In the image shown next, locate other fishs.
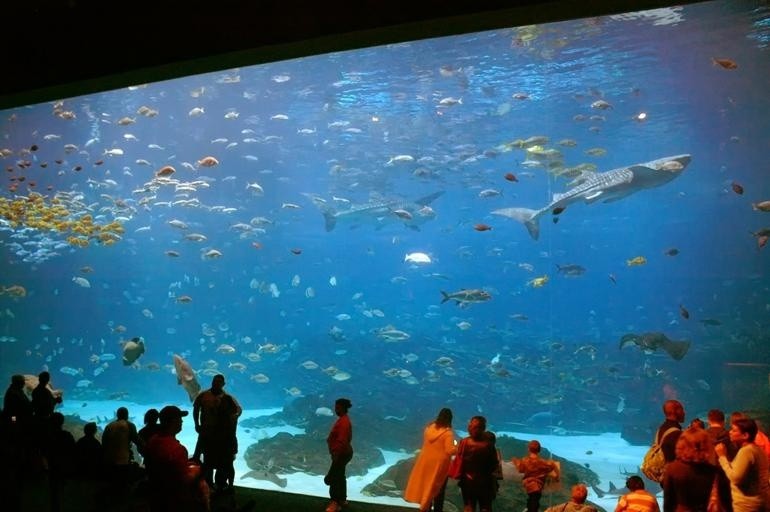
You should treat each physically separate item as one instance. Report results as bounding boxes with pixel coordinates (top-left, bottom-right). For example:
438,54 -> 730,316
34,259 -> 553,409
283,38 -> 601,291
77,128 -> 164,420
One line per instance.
0,0 -> 770,512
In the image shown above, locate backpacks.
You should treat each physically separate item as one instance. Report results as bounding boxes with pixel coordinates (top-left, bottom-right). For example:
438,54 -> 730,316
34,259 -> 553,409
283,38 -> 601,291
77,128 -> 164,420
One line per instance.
641,426 -> 681,482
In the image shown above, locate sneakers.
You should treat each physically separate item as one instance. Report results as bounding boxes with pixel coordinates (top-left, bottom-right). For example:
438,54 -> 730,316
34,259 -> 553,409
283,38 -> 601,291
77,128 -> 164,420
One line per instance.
326,500 -> 342,512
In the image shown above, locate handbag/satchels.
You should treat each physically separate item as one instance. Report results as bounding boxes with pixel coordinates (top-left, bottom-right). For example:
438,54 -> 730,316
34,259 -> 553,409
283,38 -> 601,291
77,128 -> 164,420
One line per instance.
446,457 -> 463,480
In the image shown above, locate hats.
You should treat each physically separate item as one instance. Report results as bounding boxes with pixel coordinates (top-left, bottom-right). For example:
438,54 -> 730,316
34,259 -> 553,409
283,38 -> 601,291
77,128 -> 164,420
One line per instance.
159,404 -> 189,421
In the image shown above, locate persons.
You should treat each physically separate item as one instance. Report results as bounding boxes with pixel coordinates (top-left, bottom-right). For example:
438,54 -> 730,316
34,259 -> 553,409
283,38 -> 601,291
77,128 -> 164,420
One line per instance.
641,399 -> 770,511
195,374 -> 234,491
543,484 -> 599,511
227,392 -> 243,491
485,429 -> 502,487
513,439 -> 558,511
4,375 -> 32,412
405,406 -> 460,511
457,415 -> 499,511
32,372 -> 64,415
323,398 -> 352,511
0,399 -> 211,512
614,476 -> 662,511
